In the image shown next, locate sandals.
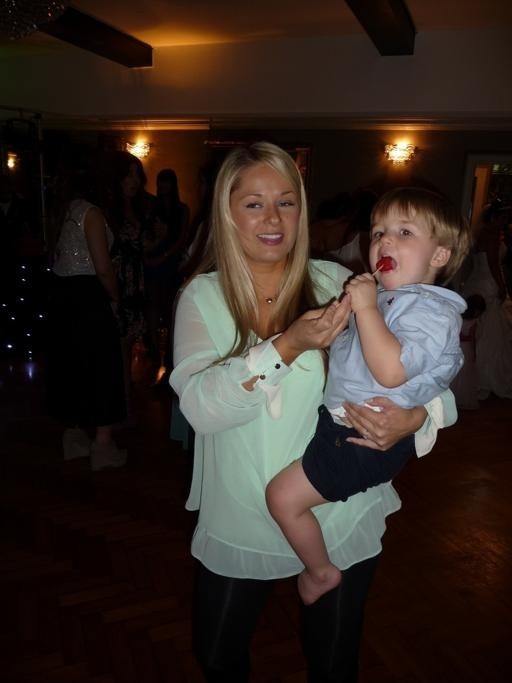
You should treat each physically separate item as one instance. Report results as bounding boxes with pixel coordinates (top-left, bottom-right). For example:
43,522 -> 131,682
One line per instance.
88,441 -> 129,473
62,427 -> 91,458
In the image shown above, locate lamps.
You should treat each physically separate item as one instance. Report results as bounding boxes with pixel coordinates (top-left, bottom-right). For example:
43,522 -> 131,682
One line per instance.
124,142 -> 150,160
383,143 -> 421,168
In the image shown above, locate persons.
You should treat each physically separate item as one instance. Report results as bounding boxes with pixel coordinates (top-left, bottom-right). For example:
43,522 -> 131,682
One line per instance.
262,183 -> 475,610
1,156 -> 511,473
163,135 -> 458,683
